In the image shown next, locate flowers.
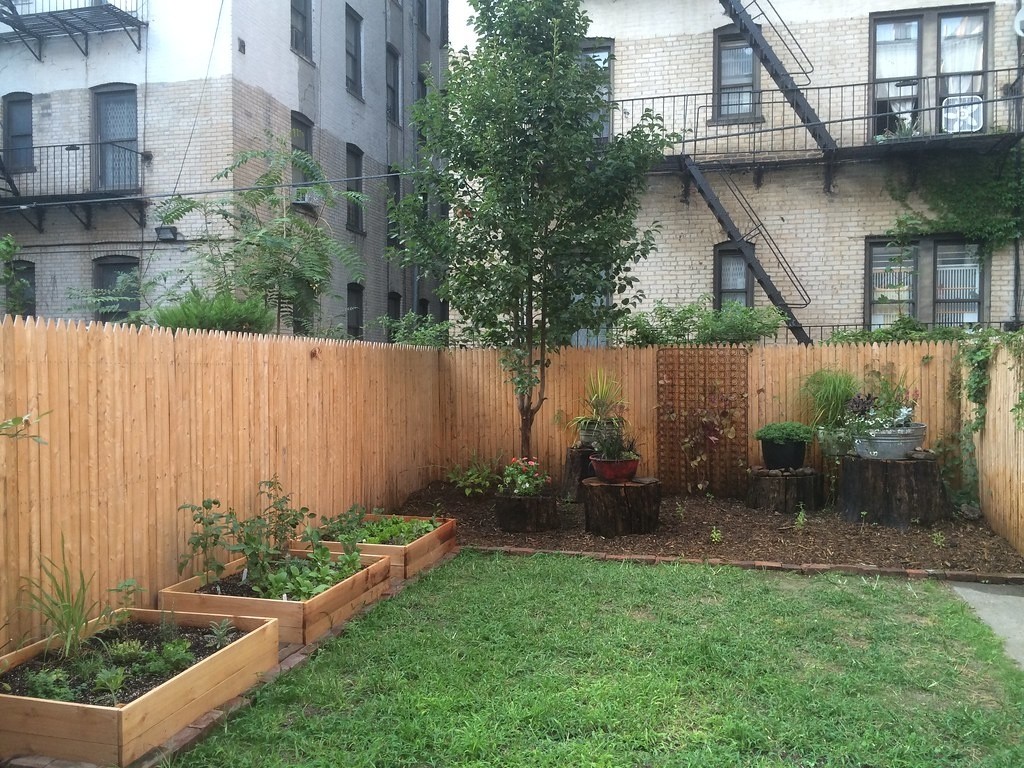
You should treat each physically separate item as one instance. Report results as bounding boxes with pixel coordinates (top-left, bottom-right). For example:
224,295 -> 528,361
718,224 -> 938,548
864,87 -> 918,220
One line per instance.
498,456 -> 553,496
821,368 -> 920,463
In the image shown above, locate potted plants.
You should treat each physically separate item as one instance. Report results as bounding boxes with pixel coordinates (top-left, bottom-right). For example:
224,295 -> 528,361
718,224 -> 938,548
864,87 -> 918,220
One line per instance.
563,366 -> 632,448
755,421 -> 812,471
883,118 -> 953,144
0,528 -> 278,768
289,502 -> 456,579
157,471 -> 391,646
790,360 -> 861,456
587,426 -> 641,484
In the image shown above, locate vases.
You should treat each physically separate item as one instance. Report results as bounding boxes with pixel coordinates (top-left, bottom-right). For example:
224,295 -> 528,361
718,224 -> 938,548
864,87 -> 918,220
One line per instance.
495,492 -> 560,531
852,423 -> 926,460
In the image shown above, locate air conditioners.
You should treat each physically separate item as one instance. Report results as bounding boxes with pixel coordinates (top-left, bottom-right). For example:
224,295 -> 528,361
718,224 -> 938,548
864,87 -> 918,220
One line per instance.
292,186 -> 320,208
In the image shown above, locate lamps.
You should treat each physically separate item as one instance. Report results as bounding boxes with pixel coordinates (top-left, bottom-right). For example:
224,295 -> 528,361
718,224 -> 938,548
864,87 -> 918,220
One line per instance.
155,226 -> 186,241
